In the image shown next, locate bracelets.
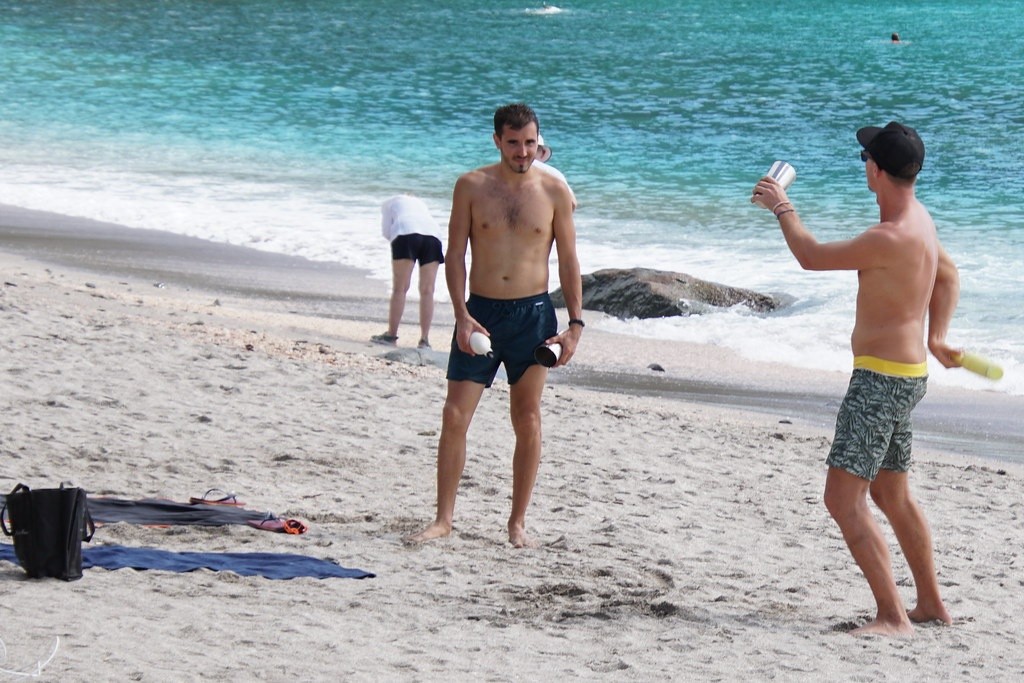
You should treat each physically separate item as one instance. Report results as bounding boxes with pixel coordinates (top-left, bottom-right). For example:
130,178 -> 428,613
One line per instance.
773,200 -> 790,214
568,319 -> 585,328
777,209 -> 795,220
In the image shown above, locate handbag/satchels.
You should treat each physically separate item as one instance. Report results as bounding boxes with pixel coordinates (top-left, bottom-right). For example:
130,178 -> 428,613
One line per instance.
0,483 -> 95,581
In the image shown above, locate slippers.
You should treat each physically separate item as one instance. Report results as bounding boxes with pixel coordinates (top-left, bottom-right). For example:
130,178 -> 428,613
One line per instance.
248,515 -> 285,532
190,489 -> 246,505
284,519 -> 307,534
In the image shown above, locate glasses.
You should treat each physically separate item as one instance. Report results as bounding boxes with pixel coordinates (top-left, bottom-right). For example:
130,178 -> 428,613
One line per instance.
861,150 -> 873,162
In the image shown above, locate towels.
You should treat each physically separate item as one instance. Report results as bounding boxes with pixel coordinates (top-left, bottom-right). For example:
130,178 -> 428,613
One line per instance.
0,494 -> 279,527
0,540 -> 377,581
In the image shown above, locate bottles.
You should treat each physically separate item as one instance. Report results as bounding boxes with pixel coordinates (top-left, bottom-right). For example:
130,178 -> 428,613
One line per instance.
948,351 -> 1004,381
470,331 -> 496,362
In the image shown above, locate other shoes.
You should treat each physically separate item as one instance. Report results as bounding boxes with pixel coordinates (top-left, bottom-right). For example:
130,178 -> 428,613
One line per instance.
370,332 -> 398,347
417,338 -> 431,349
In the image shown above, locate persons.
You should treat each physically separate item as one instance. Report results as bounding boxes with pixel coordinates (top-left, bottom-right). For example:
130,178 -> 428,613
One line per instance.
409,105 -> 582,548
751,120 -> 963,634
371,195 -> 444,352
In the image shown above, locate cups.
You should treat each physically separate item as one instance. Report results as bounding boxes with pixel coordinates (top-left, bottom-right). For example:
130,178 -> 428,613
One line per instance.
535,327 -> 565,369
756,161 -> 796,209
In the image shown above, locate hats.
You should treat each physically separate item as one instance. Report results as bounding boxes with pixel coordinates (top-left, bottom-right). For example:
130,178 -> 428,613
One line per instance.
538,135 -> 552,163
856,121 -> 925,179
